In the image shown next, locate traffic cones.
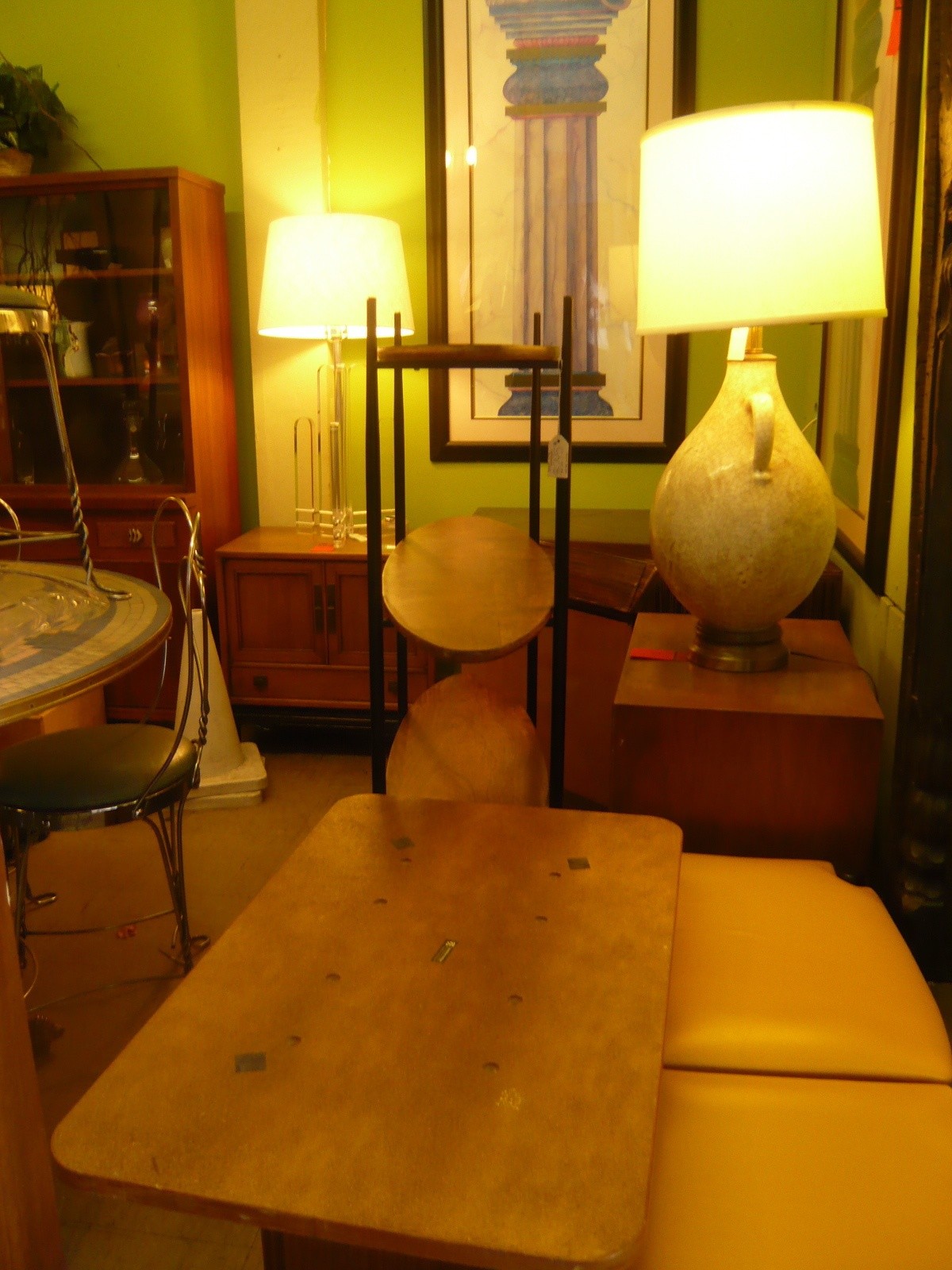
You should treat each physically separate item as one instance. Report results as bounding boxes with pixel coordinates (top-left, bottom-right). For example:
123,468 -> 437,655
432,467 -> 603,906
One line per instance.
169,609 -> 269,798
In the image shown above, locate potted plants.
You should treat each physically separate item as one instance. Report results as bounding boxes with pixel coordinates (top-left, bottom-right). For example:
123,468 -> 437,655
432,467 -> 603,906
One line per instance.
0,58 -> 79,175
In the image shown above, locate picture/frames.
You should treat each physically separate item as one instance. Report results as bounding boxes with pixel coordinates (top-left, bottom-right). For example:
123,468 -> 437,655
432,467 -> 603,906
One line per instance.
422,0 -> 702,466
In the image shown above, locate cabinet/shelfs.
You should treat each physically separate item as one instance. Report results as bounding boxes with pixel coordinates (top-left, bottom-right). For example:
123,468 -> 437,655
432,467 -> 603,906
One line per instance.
210,543 -> 430,736
608,607 -> 888,872
0,162 -> 240,728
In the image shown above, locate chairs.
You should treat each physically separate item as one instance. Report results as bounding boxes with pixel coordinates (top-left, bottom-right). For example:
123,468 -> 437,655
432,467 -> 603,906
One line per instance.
0,490 -> 220,979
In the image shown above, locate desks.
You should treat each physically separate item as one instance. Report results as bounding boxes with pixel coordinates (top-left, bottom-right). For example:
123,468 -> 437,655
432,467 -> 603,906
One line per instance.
0,557 -> 175,753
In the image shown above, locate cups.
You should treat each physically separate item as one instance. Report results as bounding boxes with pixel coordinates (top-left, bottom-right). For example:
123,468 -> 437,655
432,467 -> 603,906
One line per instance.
95,352 -> 123,378
54,320 -> 93,379
128,351 -> 149,374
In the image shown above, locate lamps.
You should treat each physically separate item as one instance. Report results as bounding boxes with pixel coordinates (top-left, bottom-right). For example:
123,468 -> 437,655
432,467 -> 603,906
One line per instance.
631,94 -> 897,677
237,202 -> 410,545
